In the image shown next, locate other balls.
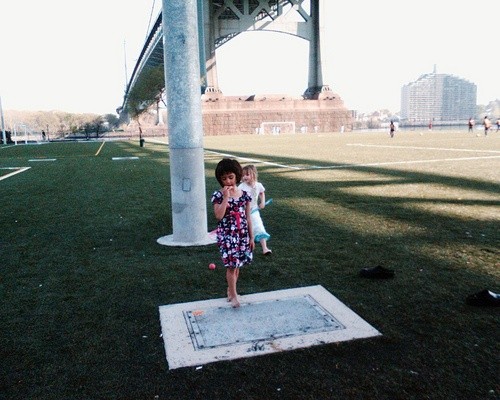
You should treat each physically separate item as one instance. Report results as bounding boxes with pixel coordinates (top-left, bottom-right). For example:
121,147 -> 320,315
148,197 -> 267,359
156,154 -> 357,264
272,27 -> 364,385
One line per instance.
209,264 -> 215,269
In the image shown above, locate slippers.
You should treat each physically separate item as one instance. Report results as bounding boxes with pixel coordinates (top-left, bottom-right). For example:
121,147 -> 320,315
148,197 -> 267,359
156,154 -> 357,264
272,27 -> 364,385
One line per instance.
360,266 -> 395,278
466,290 -> 500,307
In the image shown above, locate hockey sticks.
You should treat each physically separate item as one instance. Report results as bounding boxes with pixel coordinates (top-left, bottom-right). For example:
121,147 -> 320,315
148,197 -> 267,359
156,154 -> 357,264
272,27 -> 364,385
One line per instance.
207,198 -> 273,240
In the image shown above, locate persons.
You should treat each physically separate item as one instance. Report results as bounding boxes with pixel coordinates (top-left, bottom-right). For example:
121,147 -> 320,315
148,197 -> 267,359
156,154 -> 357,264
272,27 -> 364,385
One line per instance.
237,164 -> 272,255
496,119 -> 500,133
468,118 -> 473,133
390,120 -> 395,138
42,130 -> 46,142
482,116 -> 491,136
428,120 -> 432,132
255,124 -> 345,135
211,158 -> 254,308
